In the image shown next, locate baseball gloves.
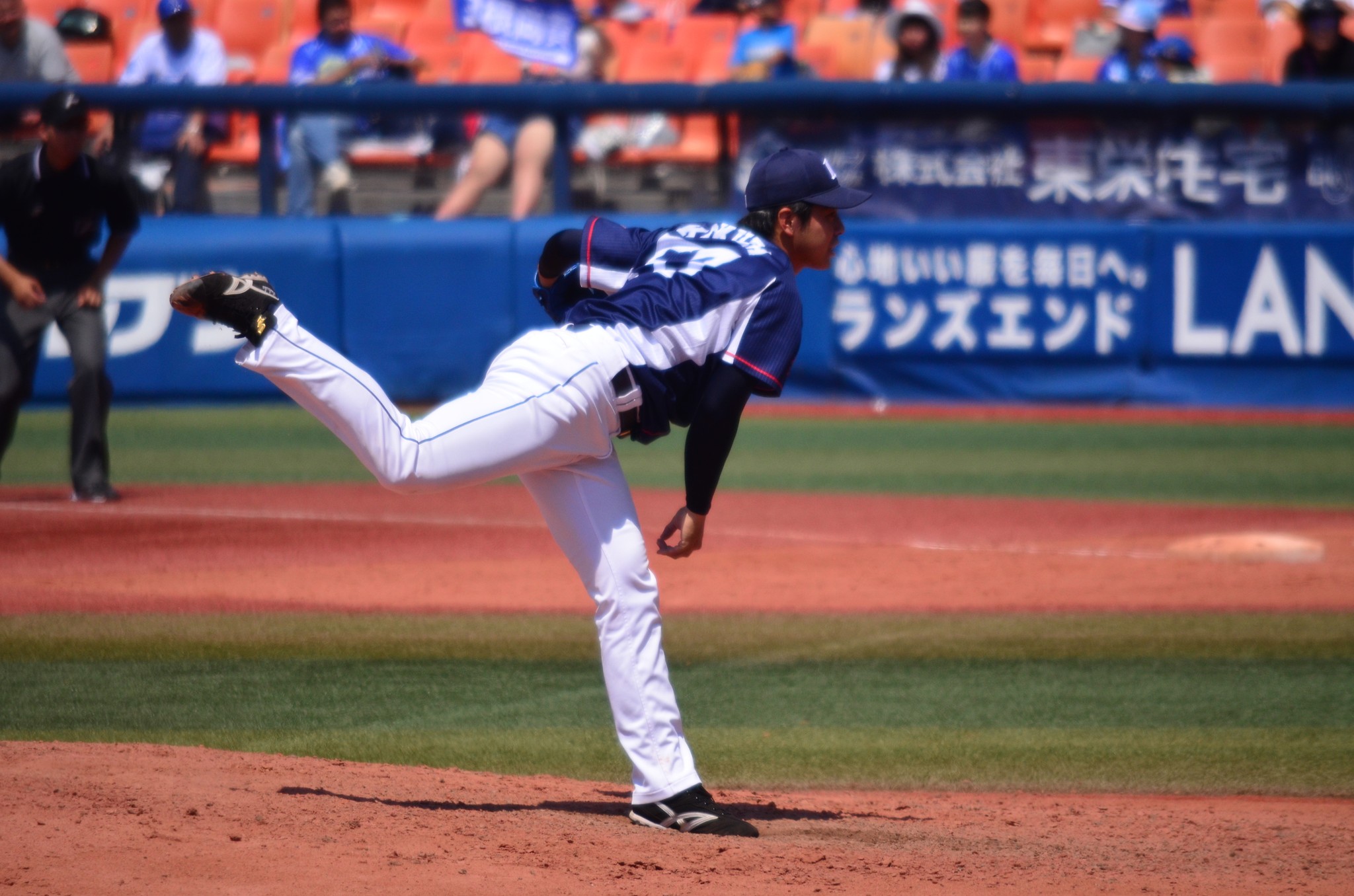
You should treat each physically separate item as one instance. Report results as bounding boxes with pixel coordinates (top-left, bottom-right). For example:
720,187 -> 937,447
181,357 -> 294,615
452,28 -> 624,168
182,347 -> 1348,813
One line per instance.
533,263 -> 609,327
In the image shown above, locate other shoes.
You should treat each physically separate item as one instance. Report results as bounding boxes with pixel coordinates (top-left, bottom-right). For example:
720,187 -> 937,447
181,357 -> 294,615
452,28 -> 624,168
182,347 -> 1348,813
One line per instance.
79,484 -> 119,503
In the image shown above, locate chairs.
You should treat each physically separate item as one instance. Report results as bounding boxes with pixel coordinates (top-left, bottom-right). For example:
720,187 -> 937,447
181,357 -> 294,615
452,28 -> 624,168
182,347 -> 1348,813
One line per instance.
8,0 -> 1353,219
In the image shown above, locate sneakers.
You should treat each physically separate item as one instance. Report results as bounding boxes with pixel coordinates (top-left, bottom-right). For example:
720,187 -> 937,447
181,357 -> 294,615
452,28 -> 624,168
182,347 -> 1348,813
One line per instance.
170,271 -> 283,345
626,776 -> 758,838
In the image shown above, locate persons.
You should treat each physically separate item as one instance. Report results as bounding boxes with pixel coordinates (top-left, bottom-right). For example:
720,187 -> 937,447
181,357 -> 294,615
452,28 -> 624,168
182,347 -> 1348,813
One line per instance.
0,94 -> 156,499
168,150 -> 871,840
1,0 -> 1354,219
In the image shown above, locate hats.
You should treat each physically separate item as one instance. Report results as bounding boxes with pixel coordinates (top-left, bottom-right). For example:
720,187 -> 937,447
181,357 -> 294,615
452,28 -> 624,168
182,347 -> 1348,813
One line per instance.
161,0 -> 191,19
887,1 -> 946,45
1114,1 -> 1159,33
1146,38 -> 1193,62
743,148 -> 878,210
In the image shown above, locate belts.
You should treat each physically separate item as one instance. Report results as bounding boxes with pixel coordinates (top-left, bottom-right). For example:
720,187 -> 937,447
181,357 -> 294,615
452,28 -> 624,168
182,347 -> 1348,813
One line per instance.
610,368 -> 637,441
